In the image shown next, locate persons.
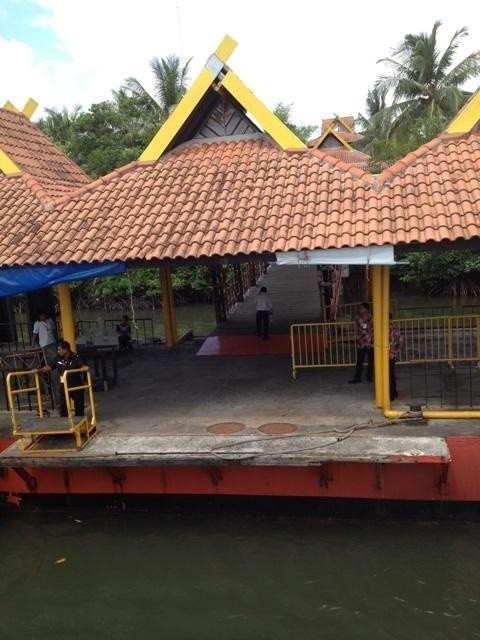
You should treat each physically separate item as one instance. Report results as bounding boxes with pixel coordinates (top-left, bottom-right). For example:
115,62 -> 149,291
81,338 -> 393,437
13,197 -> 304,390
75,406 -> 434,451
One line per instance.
348,302 -> 374,384
31,306 -> 56,348
32,341 -> 85,416
116,315 -> 131,352
389,312 -> 405,402
255,287 -> 273,340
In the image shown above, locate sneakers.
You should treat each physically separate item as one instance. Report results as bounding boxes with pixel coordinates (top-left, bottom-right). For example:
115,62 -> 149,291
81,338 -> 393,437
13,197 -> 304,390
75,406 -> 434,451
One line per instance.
348,379 -> 361,384
